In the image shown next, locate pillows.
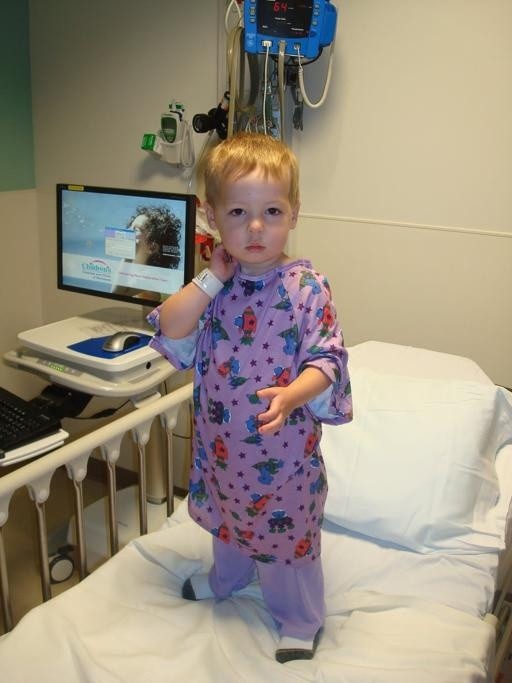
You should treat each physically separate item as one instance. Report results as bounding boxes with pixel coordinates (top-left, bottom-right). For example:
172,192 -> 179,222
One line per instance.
318,341 -> 499,549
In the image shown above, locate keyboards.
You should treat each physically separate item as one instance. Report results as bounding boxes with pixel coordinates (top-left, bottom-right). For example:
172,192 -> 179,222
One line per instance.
0,387 -> 60,453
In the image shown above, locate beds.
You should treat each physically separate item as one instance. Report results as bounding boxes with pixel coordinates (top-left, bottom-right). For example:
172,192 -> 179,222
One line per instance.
2,340 -> 510,683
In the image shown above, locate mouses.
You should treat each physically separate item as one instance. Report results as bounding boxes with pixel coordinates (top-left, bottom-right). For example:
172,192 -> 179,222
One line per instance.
102,331 -> 141,353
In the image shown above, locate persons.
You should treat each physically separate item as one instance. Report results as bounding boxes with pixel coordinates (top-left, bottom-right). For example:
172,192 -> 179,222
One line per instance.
111,204 -> 182,303
145,129 -> 354,664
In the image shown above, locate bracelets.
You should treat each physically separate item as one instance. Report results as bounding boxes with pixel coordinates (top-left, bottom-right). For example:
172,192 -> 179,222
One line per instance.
191,266 -> 225,300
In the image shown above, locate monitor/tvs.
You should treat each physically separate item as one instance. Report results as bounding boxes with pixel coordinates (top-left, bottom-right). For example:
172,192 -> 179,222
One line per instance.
56,183 -> 195,308
